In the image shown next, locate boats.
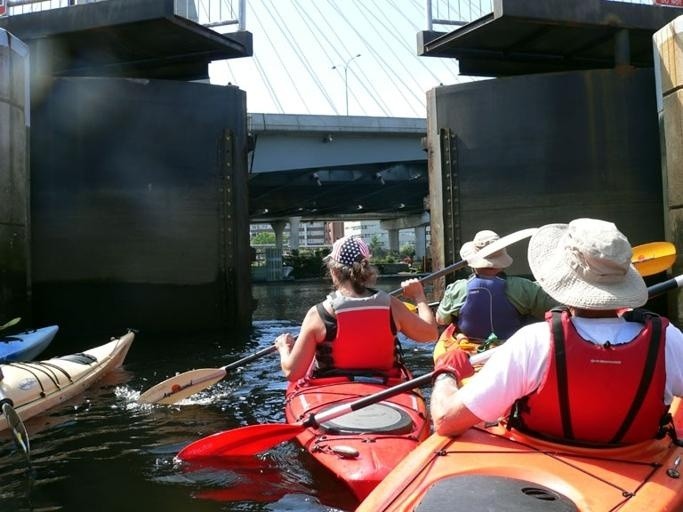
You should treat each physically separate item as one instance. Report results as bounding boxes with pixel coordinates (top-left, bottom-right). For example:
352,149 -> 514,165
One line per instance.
287,365 -> 430,506
433,321 -> 506,367
1,324 -> 135,432
354,397 -> 683,512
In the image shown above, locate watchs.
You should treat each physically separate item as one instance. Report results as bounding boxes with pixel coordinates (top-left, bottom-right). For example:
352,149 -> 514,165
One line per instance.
432,371 -> 457,387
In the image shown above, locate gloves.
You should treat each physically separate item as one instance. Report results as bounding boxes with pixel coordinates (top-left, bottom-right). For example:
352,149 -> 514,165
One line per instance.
429,349 -> 476,387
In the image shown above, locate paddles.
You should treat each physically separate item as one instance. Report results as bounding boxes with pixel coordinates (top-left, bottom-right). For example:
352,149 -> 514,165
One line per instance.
173,344 -> 503,459
402,240 -> 676,313
0,317 -> 22,331
0,381 -> 31,468
137,227 -> 538,406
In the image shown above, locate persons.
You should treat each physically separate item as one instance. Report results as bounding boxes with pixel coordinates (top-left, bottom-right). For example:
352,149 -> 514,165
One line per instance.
432,228 -> 564,344
429,216 -> 682,450
273,235 -> 440,385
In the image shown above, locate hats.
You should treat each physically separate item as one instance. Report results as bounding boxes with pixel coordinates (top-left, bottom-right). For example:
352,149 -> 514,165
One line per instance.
321,235 -> 372,267
459,228 -> 515,271
526,217 -> 650,311
